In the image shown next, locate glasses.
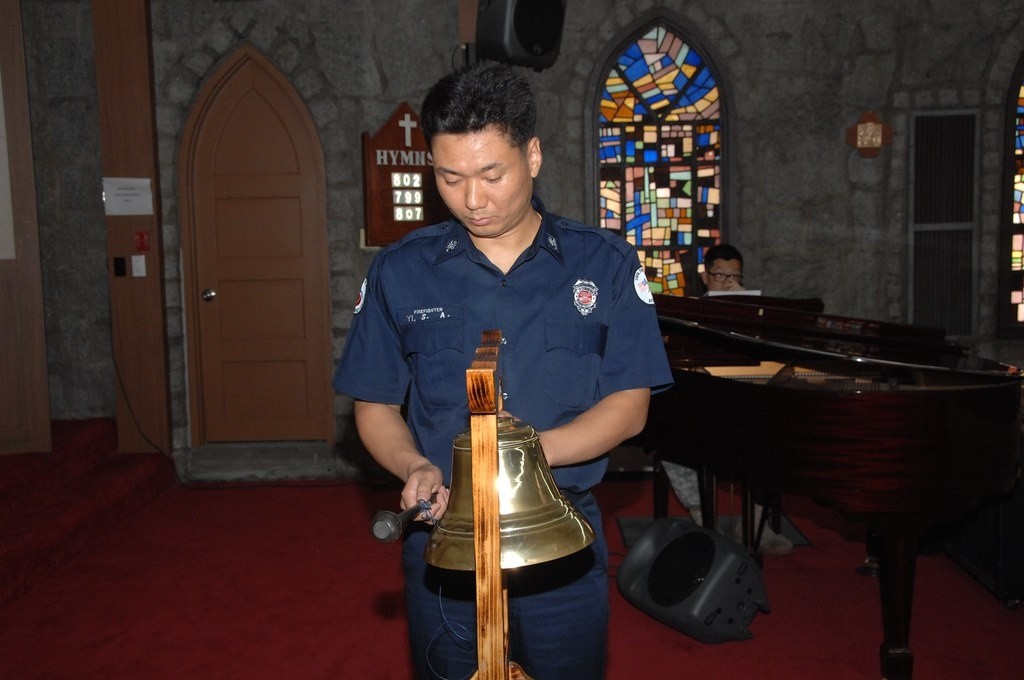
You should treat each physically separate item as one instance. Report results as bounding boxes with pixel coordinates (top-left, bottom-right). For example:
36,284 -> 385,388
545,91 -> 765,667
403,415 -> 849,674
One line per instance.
705,270 -> 743,283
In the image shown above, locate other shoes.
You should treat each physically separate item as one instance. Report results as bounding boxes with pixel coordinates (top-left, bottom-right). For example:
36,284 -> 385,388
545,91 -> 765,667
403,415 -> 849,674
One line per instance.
733,514 -> 793,556
690,509 -> 725,536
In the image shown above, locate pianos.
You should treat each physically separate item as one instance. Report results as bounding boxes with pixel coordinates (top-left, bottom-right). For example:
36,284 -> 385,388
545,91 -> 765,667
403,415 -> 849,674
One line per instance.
621,289 -> 1024,680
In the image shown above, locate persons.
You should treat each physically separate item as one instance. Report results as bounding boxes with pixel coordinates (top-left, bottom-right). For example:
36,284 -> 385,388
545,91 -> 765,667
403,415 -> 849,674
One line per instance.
699,242 -> 747,295
331,61 -> 674,680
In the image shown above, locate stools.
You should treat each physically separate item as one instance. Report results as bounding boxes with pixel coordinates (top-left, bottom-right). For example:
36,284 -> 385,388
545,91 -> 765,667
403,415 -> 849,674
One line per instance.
653,454 -> 782,556
